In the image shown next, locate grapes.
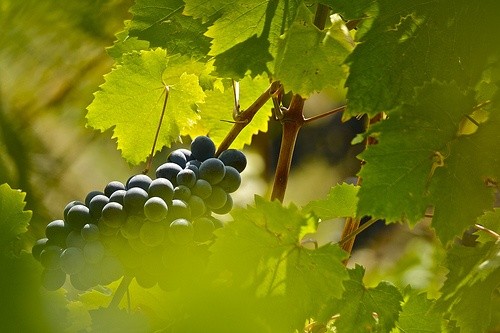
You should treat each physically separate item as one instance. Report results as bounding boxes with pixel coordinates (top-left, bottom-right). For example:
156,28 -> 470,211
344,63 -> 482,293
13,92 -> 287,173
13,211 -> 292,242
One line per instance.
460,228 -> 479,247
31,135 -> 246,289
269,111 -> 385,252
